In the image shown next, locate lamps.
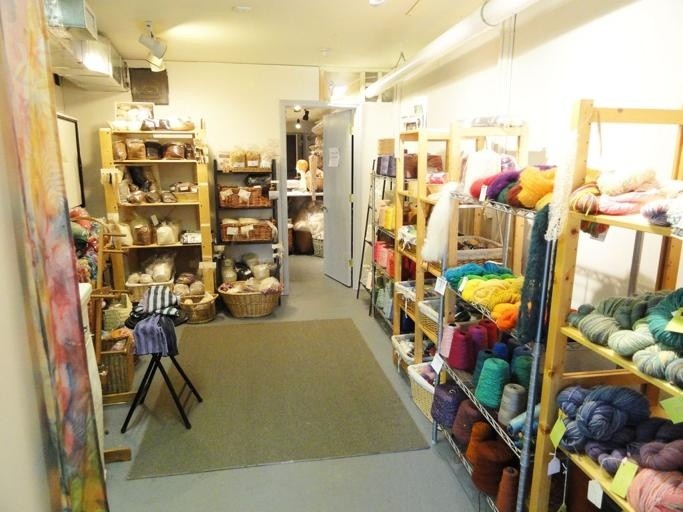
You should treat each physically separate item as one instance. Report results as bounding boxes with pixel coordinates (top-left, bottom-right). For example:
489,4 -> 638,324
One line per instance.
137,20 -> 170,74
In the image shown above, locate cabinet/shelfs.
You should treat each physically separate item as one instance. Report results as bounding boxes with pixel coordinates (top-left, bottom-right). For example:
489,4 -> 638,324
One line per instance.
99,129 -> 281,305
359,99 -> 683,512
46,0 -> 129,92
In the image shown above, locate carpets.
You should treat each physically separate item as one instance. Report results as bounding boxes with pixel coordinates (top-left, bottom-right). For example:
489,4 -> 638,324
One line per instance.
126,319 -> 430,479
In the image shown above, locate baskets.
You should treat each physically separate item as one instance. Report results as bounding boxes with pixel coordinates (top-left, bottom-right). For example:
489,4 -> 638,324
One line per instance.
100,293 -> 134,394
396,280 -> 447,336
407,362 -> 447,432
399,224 -> 503,273
124,268 -> 176,303
288,227 -> 324,257
218,280 -> 281,318
216,182 -> 274,209
111,100 -> 155,133
392,332 -> 440,377
219,217 -> 277,242
179,294 -> 218,325
305,174 -> 324,191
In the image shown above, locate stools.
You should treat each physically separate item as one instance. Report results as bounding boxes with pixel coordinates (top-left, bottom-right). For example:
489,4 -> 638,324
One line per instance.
121,309 -> 203,433
